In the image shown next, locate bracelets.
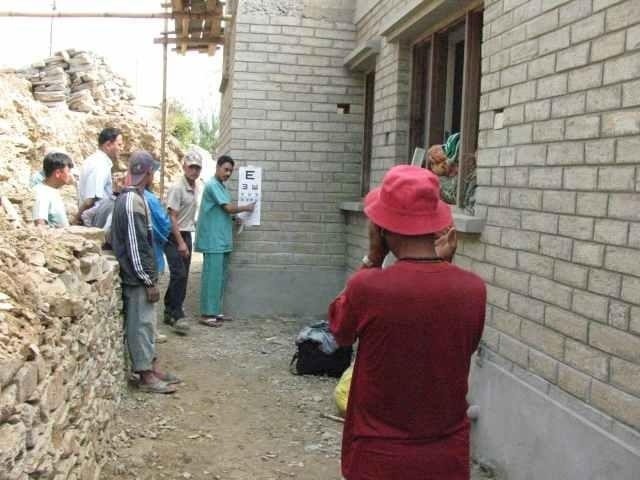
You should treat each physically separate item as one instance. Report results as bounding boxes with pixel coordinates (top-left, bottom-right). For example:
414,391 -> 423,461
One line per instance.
360,255 -> 383,269
236,207 -> 240,213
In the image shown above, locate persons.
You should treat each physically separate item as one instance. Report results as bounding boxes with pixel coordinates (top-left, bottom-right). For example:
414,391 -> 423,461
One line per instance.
70,127 -> 123,224
29,152 -> 74,227
164,151 -> 202,334
110,151 -> 185,395
32,149 -> 171,344
194,156 -> 255,327
325,161 -> 488,480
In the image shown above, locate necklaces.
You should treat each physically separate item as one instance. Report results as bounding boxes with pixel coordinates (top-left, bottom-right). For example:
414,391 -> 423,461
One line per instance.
399,255 -> 445,263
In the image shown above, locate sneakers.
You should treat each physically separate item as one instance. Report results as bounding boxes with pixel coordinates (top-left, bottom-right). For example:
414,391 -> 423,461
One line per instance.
156,333 -> 166,342
165,317 -> 190,332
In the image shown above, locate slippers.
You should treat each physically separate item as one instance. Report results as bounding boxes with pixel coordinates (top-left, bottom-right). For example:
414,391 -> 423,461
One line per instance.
197,312 -> 232,326
137,372 -> 181,394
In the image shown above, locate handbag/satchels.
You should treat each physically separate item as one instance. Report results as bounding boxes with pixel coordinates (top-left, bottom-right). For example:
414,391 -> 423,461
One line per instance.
296,340 -> 353,376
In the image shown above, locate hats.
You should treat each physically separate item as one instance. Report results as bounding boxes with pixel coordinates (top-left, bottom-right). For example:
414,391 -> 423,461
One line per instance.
184,151 -> 203,168
127,150 -> 160,186
364,164 -> 452,235
